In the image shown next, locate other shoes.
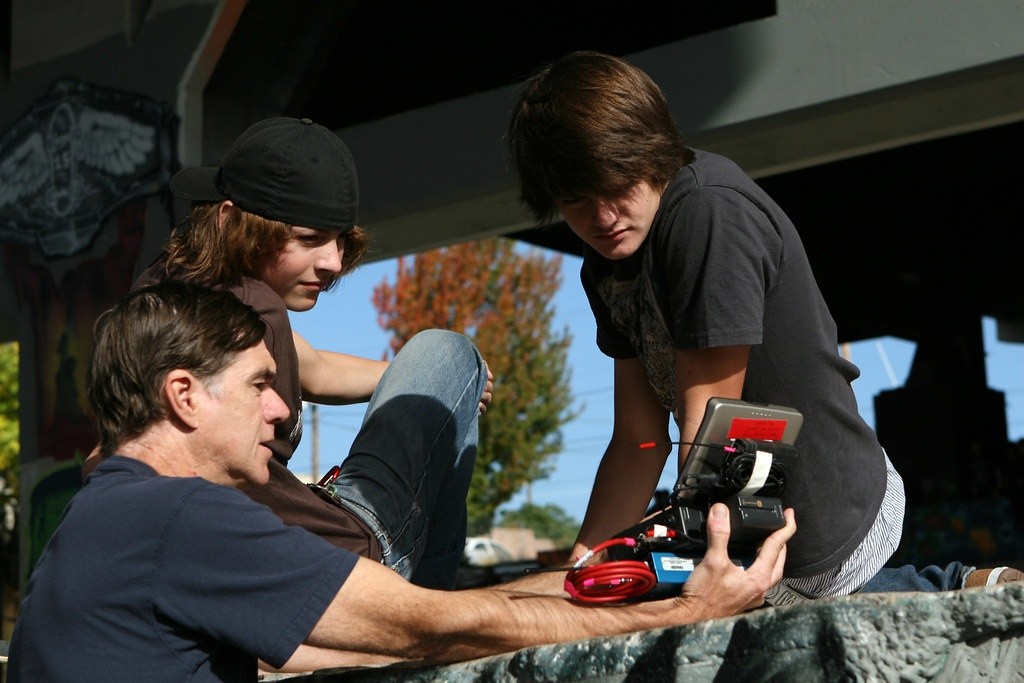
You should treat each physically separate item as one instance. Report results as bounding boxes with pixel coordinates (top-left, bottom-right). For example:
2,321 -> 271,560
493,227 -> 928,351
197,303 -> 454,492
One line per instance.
964,565 -> 1024,589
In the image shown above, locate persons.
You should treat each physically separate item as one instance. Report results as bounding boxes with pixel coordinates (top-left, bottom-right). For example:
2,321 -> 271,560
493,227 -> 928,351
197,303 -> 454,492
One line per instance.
6,51 -> 1024,683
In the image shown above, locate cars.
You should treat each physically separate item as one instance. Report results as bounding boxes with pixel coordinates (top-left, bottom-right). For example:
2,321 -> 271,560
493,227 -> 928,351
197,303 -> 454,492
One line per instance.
454,537 -> 513,568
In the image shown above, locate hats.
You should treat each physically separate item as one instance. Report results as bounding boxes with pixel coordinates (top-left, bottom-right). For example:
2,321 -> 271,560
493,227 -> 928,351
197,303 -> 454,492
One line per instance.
213,116 -> 358,231
